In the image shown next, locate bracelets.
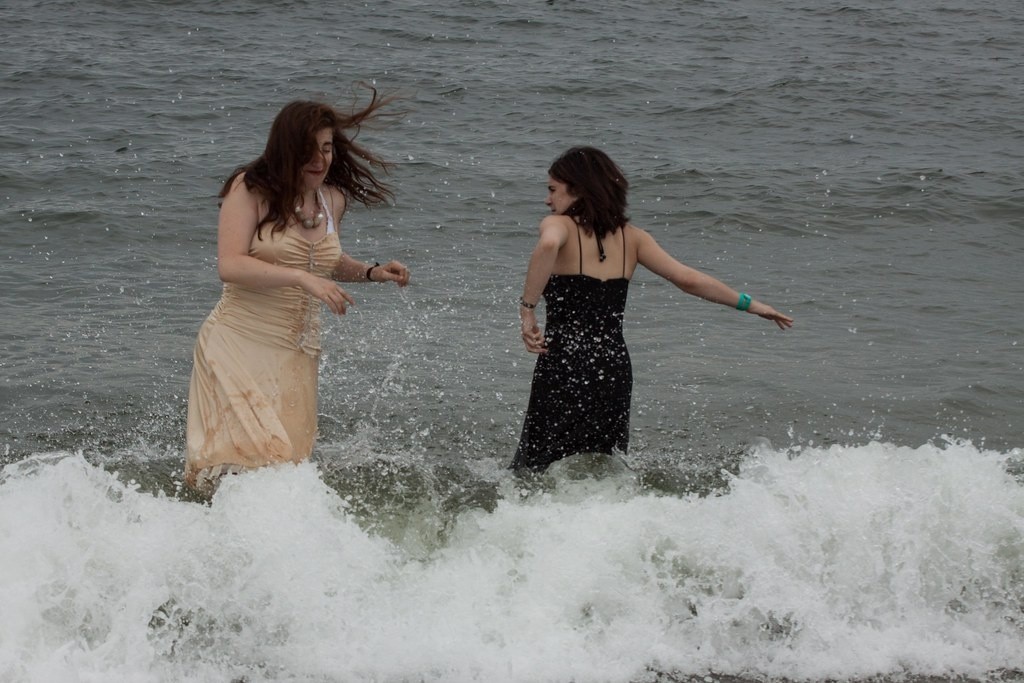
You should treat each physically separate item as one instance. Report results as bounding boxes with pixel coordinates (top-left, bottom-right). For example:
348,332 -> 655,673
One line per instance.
519,295 -> 536,309
736,293 -> 744,311
366,262 -> 380,282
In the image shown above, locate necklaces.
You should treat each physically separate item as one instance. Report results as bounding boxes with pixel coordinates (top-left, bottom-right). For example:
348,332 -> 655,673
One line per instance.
291,188 -> 323,228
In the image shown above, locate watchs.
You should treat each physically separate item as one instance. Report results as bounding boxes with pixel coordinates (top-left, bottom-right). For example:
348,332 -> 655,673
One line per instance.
741,293 -> 751,311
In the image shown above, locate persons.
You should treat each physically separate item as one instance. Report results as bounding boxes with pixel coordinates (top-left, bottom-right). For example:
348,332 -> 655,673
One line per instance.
508,147 -> 794,469
184,79 -> 411,467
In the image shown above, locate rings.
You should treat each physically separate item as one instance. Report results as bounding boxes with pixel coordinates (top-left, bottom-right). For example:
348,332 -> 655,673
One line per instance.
327,288 -> 337,296
